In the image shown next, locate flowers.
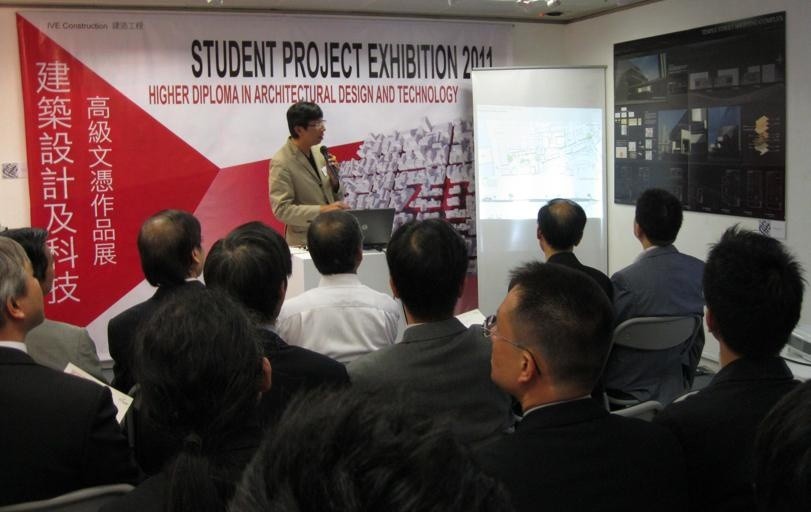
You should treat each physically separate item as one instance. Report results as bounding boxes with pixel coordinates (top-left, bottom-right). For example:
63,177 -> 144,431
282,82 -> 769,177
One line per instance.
320,146 -> 339,179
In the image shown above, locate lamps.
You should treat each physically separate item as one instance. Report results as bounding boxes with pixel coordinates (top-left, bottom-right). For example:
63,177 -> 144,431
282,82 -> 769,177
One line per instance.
127,383 -> 149,449
609,400 -> 666,425
0,483 -> 137,512
595,316 -> 703,412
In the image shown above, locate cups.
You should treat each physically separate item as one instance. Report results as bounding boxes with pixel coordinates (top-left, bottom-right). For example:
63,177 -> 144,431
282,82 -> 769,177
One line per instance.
481,315 -> 542,377
308,120 -> 327,127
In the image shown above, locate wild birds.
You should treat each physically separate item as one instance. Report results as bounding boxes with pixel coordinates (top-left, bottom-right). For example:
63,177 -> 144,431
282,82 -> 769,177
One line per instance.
344,208 -> 395,249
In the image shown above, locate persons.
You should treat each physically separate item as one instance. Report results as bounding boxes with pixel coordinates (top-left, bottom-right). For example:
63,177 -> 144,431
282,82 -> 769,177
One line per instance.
755,380 -> 809,509
1,236 -> 133,506
203,220 -> 351,469
231,385 -> 514,510
270,100 -> 352,250
272,209 -> 406,371
106,284 -> 271,509
591,189 -> 705,410
537,198 -> 613,302
347,218 -> 519,451
472,263 -> 692,509
0,227 -> 109,384
650,222 -> 809,508
108,209 -> 204,395
